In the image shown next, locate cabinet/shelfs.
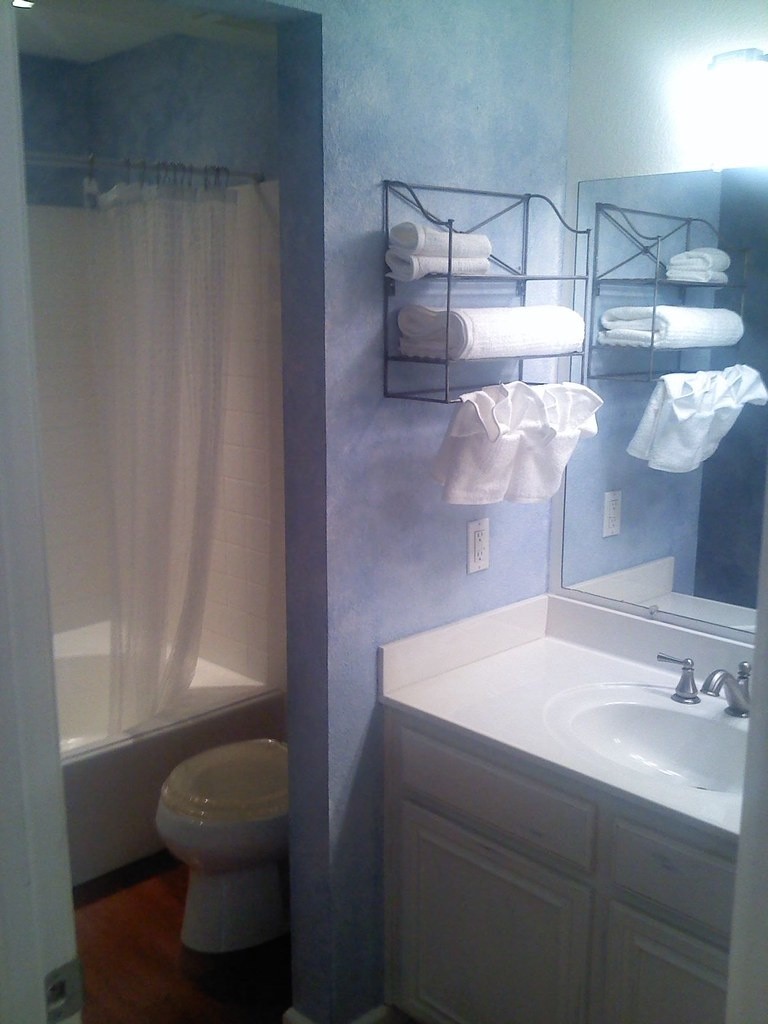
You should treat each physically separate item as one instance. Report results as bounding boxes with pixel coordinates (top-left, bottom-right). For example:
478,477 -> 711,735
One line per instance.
380,704 -> 739,1024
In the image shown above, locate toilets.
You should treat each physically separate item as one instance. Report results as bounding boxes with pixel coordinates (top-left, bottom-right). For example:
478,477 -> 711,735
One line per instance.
154,734 -> 294,954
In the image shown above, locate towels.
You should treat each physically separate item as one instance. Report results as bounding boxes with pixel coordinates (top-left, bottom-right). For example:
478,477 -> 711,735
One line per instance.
597,305 -> 746,349
625,360 -> 768,474
663,246 -> 731,290
397,303 -> 585,361
380,219 -> 494,281
427,378 -> 605,508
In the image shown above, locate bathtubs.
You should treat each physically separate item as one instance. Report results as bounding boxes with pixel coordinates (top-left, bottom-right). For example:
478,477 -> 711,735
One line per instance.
53,615 -> 268,887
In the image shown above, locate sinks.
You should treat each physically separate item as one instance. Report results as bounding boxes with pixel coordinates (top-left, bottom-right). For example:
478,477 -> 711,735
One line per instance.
546,676 -> 751,796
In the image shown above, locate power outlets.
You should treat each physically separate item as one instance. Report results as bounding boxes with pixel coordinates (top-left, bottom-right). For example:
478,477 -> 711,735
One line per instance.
467,516 -> 491,573
602,490 -> 622,538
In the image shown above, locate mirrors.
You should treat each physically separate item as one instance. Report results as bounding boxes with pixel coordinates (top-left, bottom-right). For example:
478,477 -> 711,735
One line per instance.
560,166 -> 768,635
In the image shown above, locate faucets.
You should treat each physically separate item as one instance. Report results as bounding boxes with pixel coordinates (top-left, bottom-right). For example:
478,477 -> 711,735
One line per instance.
698,659 -> 752,719
